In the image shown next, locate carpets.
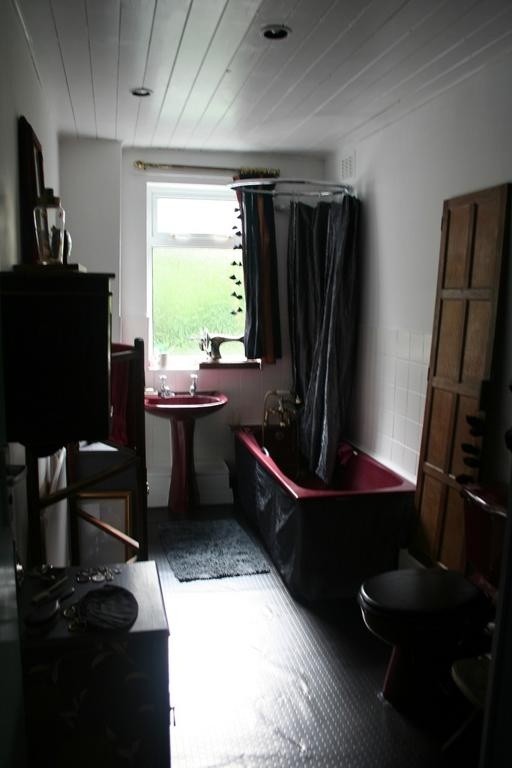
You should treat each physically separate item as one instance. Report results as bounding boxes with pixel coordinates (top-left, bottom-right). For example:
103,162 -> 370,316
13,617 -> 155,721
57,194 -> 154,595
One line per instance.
158,518 -> 270,581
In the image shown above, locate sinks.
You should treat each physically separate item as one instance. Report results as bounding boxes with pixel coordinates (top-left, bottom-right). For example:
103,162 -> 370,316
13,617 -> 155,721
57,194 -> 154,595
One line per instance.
144,391 -> 229,417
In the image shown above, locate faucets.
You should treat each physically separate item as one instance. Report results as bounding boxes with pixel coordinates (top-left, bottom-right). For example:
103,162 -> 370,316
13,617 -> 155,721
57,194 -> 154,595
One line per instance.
190,373 -> 197,397
281,400 -> 300,426
160,375 -> 171,398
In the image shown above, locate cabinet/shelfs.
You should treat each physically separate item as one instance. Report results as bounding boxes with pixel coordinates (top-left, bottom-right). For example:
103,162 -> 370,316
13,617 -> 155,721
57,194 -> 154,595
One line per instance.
0,271 -> 115,444
18,560 -> 170,768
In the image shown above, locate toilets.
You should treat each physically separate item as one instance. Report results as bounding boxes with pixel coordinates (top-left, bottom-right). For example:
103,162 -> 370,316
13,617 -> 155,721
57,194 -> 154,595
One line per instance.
359,476 -> 511,729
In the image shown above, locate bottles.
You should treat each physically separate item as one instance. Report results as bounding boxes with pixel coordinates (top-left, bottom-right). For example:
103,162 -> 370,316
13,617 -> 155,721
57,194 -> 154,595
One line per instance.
33,187 -> 65,266
53,227 -> 72,264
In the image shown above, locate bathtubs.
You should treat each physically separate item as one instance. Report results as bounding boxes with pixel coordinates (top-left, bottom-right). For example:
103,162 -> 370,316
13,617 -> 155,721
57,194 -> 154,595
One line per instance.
233,420 -> 417,606
